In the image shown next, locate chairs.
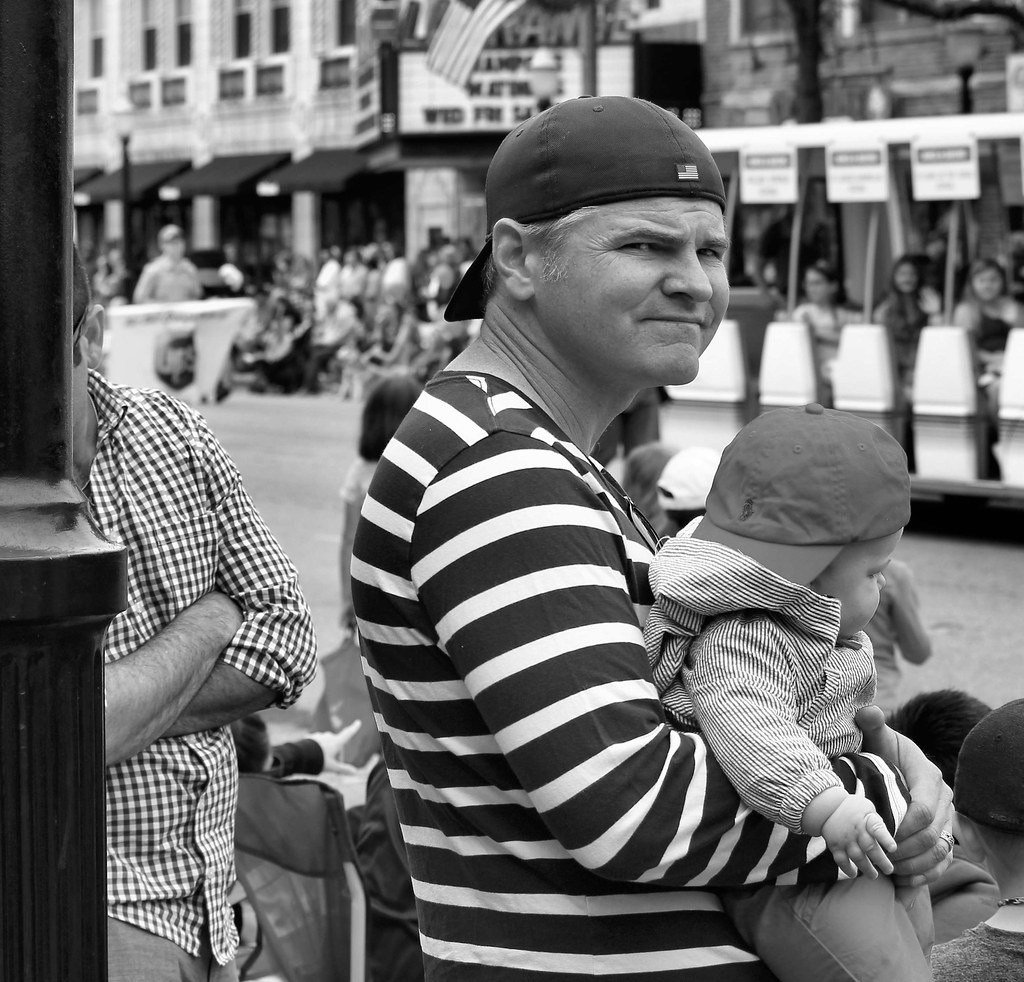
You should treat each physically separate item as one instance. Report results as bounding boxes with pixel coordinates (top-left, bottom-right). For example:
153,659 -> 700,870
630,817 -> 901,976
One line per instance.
226,763 -> 424,982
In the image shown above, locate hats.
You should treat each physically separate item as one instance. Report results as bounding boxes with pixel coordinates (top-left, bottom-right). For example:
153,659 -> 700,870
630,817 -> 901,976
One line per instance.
445,93 -> 726,322
954,698 -> 1024,831
691,402 -> 912,586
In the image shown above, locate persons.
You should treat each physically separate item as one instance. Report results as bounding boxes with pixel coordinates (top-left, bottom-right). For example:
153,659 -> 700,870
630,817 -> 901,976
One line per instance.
70,225 -> 1024,981
349,95 -> 959,982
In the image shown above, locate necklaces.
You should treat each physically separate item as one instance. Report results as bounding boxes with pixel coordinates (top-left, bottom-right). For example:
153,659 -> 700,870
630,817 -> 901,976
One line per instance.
997,897 -> 1024,906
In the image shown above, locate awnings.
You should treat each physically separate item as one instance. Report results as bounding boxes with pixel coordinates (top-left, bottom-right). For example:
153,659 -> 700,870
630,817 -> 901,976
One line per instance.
68,149 -> 374,205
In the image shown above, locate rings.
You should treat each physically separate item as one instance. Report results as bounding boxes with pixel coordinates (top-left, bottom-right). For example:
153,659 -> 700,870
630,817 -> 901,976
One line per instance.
937,830 -> 956,854
